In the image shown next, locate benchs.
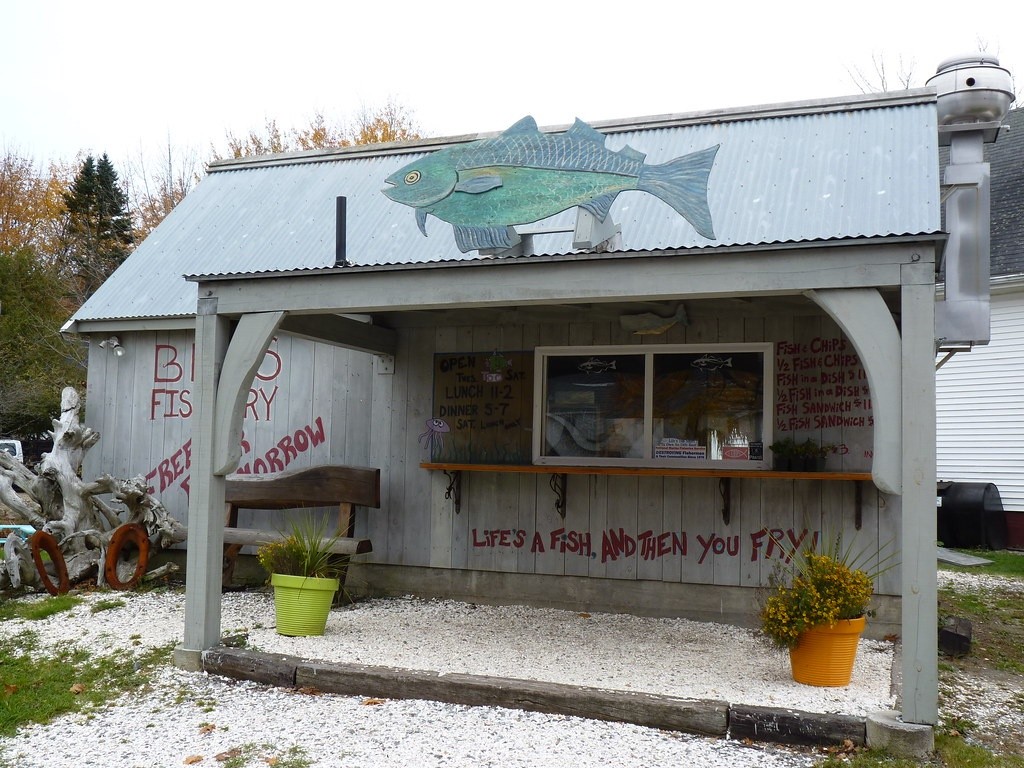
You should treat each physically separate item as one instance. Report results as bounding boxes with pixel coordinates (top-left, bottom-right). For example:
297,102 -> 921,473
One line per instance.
222,463 -> 381,602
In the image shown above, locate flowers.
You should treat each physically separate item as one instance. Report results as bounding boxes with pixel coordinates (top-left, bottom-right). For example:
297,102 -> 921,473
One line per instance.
256,502 -> 360,607
747,514 -> 902,656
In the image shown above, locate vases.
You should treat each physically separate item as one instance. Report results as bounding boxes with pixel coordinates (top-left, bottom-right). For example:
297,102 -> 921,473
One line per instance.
789,617 -> 864,687
271,573 -> 339,636
